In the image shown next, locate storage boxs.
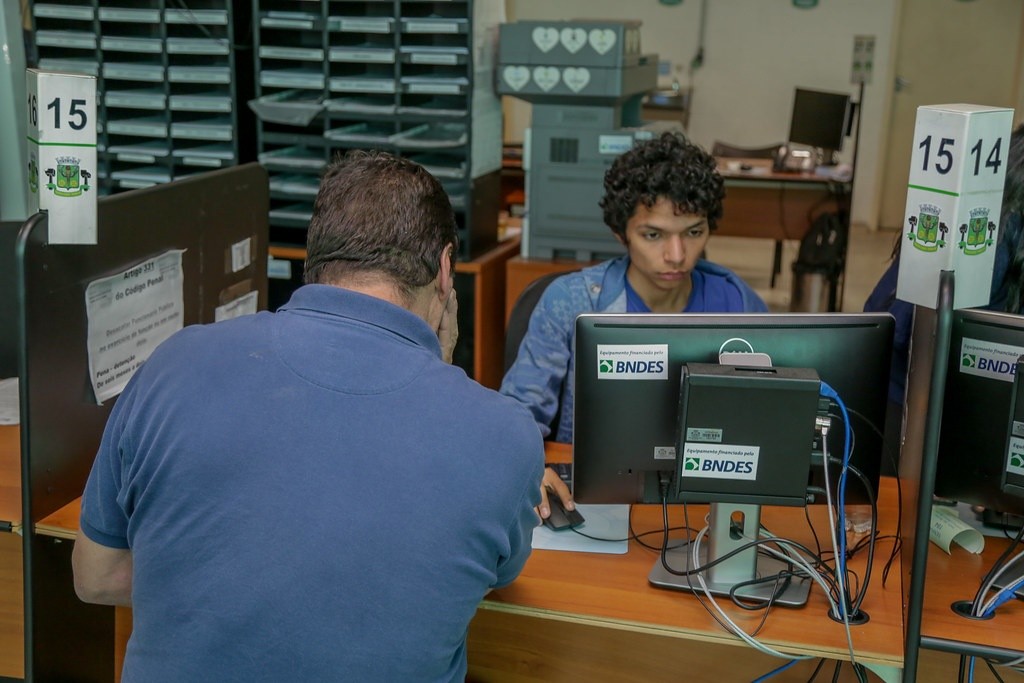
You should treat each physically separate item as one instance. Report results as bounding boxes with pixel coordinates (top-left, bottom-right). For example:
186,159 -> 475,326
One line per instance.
27,69 -> 97,245
896,103 -> 1014,309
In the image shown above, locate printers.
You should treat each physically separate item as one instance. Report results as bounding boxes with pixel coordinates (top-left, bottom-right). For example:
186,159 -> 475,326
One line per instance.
497,18 -> 689,267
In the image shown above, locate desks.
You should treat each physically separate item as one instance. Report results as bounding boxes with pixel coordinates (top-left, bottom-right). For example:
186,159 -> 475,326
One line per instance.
899,477 -> 1024,683
714,156 -> 852,310
1,163 -> 905,683
268,215 -> 522,392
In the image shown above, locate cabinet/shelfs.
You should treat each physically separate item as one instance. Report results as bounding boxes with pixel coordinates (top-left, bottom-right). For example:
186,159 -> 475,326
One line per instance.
27,0 -> 506,263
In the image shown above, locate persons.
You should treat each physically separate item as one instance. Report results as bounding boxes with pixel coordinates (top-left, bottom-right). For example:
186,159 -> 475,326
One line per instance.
71,151 -> 547,683
497,131 -> 771,526
863,123 -> 1024,416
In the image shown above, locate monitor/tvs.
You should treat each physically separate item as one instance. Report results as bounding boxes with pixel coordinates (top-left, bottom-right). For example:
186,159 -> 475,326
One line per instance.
930,308 -> 1024,600
570,313 -> 896,608
787,87 -> 851,167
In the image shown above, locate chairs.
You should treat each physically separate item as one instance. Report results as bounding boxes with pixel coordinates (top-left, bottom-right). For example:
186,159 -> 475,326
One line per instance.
712,141 -> 790,288
506,269 -> 584,371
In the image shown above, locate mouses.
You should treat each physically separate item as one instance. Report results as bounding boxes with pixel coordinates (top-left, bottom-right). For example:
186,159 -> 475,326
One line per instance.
538,490 -> 585,531
741,163 -> 753,170
